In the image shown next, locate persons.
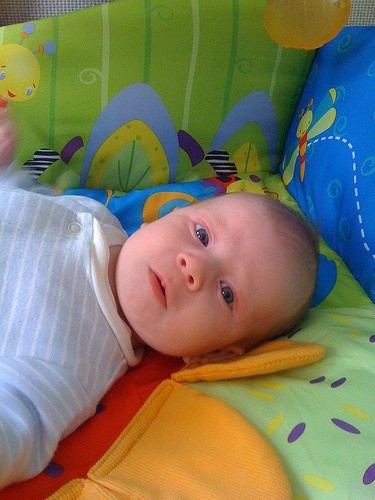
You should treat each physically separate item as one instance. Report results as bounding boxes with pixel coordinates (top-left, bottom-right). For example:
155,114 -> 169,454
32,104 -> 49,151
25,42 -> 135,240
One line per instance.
0,178 -> 320,491
